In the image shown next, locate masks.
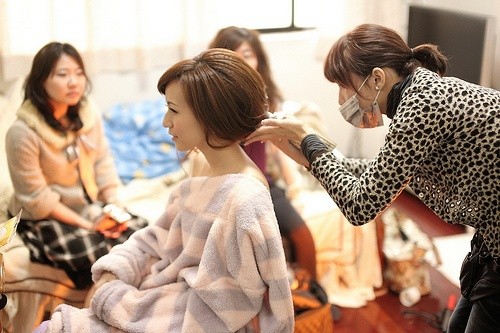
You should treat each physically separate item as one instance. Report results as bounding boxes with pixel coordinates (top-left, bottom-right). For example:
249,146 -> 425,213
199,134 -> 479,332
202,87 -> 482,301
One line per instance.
339,73 -> 384,128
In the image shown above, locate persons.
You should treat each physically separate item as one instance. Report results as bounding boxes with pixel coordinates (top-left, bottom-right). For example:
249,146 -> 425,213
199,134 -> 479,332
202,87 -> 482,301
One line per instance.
5,43 -> 150,291
243,22 -> 500,333
34,49 -> 292,333
207,26 -> 328,305
